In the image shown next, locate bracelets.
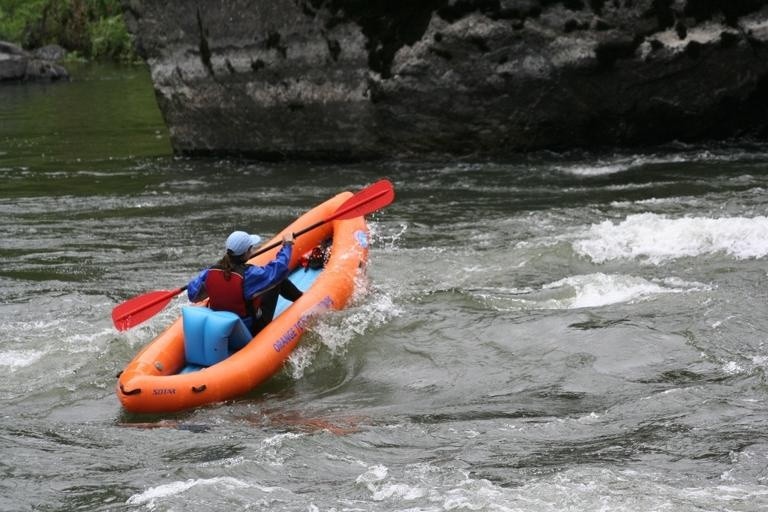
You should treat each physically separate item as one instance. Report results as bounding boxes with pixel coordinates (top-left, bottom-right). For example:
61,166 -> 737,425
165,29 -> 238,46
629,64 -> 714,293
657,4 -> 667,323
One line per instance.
282,240 -> 295,246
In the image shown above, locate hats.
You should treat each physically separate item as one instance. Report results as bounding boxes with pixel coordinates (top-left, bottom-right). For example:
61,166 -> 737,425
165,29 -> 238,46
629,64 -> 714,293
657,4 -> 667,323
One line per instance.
225,230 -> 261,256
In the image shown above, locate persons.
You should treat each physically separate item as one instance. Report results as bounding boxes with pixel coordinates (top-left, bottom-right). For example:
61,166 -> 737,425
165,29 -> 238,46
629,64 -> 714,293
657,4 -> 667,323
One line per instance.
188,231 -> 303,337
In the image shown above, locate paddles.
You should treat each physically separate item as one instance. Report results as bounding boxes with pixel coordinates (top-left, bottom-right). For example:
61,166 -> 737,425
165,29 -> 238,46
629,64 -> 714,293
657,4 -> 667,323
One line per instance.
112,180 -> 395,332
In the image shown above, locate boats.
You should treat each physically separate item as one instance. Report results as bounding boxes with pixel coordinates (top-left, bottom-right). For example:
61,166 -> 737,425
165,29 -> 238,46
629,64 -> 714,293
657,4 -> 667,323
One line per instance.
113,190 -> 373,414
